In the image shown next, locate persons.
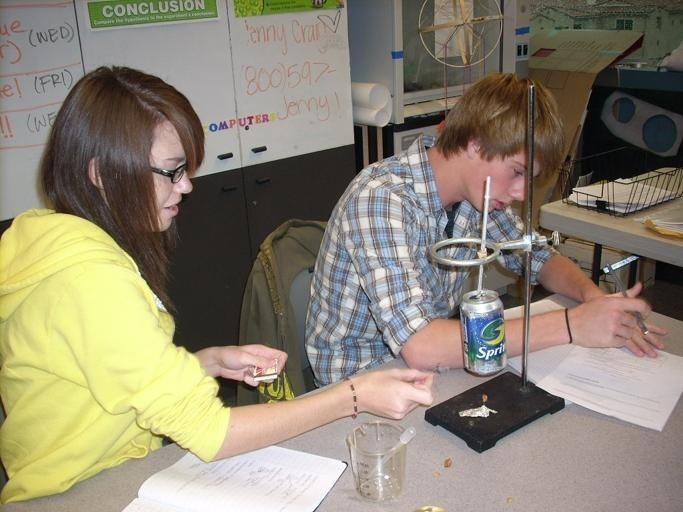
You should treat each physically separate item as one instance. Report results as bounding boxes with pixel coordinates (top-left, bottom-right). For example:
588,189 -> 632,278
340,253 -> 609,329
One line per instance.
302,73 -> 668,390
1,64 -> 436,503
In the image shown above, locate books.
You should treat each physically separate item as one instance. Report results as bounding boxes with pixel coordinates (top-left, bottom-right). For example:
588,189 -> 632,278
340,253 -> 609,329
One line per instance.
122,444 -> 348,512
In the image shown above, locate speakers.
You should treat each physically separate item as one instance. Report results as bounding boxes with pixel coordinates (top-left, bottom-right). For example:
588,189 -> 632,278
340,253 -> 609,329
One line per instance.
601,92 -> 683,158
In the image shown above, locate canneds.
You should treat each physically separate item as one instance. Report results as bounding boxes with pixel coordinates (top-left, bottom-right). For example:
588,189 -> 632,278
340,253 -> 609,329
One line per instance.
461,290 -> 508,377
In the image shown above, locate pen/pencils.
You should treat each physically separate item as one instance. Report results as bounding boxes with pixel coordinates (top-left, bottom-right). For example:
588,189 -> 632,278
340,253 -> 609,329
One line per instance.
607,263 -> 648,335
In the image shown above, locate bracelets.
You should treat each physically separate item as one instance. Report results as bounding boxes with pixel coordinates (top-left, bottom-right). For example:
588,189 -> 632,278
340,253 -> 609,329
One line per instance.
345,378 -> 357,419
564,308 -> 572,343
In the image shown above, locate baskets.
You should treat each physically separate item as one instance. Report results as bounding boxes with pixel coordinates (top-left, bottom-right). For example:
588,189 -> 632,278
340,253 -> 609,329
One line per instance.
558,146 -> 682,218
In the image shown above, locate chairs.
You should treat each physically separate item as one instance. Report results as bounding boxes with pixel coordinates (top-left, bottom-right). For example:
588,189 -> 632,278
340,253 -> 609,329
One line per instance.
234,217 -> 333,408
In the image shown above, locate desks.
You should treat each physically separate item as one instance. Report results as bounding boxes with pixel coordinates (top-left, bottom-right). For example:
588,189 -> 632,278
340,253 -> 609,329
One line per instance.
537,165 -> 681,295
0,289 -> 683,512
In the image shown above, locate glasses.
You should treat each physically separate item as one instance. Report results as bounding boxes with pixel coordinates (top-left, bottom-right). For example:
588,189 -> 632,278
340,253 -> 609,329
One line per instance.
150,164 -> 189,183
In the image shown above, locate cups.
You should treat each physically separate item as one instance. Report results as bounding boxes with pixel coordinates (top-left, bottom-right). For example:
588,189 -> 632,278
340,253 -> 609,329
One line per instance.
346,421 -> 411,503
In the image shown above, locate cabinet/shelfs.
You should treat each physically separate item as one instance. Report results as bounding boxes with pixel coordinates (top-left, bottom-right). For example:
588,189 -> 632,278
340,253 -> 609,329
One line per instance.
0,0 -> 91,223
241,144 -> 357,286
148,167 -> 250,449
71,0 -> 241,191
222,0 -> 356,172
